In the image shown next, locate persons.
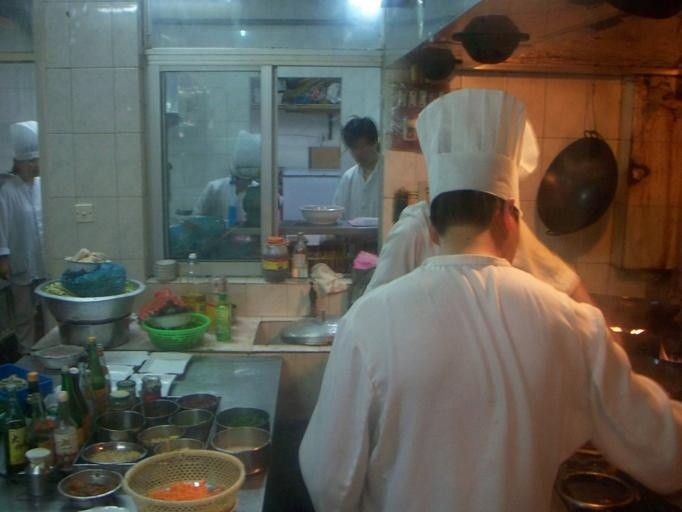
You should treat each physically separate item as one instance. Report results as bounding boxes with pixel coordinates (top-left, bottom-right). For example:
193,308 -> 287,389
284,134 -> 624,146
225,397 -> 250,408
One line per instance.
332,118 -> 383,226
357,198 -> 598,305
194,169 -> 283,222
296,190 -> 680,511
0,158 -> 59,355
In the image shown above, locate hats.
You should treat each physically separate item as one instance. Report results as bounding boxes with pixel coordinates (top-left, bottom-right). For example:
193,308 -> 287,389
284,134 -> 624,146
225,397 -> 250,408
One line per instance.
229,130 -> 262,181
10,120 -> 40,161
414,88 -> 538,212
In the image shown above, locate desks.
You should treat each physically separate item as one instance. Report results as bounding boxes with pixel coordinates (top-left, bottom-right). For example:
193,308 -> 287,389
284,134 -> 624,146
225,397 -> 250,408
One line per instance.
0,350 -> 284,511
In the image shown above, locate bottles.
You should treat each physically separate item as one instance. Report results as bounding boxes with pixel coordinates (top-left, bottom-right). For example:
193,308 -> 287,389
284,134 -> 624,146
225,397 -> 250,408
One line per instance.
185,252 -> 199,283
181,275 -> 233,344
292,230 -> 311,280
392,86 -> 453,149
1,336 -> 112,500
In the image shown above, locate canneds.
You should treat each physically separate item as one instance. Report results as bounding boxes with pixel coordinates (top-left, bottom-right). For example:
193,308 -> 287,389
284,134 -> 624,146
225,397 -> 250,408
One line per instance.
107,390 -> 130,411
116,379 -> 136,402
140,375 -> 161,399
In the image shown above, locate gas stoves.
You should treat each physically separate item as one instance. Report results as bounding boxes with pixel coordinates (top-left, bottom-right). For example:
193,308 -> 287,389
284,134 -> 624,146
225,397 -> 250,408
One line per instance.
606,316 -> 659,353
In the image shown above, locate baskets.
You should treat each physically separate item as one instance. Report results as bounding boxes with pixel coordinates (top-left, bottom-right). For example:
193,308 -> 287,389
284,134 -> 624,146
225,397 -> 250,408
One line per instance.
142,312 -> 210,350
123,449 -> 245,512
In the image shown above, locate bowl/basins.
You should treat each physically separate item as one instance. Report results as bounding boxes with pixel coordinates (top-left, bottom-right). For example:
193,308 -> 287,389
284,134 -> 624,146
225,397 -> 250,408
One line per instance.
57,375 -> 274,512
555,471 -> 638,512
298,202 -> 346,227
31,276 -> 150,326
151,257 -> 180,280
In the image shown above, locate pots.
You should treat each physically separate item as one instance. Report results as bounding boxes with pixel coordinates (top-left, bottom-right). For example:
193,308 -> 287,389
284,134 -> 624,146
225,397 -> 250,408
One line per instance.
588,293 -> 663,322
535,129 -> 617,234
53,312 -> 138,351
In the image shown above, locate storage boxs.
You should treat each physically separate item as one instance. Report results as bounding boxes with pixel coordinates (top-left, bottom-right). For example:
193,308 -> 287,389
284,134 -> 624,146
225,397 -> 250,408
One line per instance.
308,146 -> 340,170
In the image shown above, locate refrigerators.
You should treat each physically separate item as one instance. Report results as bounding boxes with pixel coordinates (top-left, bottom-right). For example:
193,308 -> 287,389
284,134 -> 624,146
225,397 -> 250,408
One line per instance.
281,168 -> 344,221
